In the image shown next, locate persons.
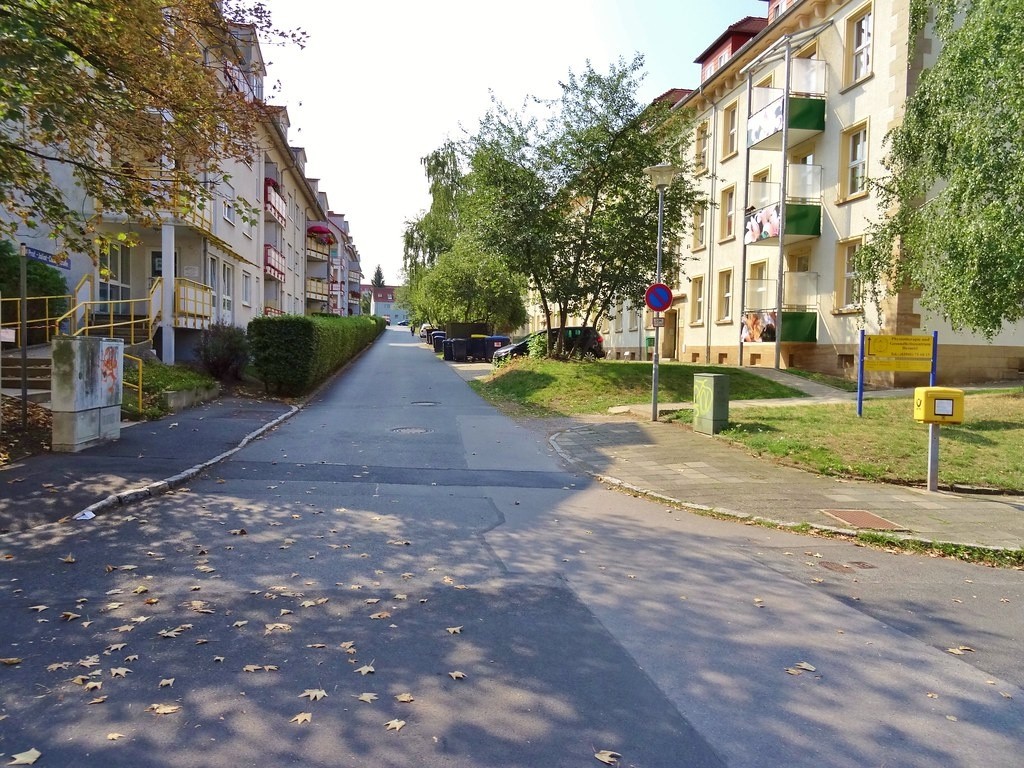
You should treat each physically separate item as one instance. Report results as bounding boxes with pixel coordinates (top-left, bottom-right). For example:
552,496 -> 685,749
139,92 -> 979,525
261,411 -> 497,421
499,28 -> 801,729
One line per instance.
410,324 -> 415,336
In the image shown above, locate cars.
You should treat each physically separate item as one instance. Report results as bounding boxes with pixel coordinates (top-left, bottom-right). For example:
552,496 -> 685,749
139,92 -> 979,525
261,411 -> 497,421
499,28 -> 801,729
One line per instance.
400,320 -> 411,326
382,316 -> 391,326
493,327 -> 605,368
420,324 -> 436,338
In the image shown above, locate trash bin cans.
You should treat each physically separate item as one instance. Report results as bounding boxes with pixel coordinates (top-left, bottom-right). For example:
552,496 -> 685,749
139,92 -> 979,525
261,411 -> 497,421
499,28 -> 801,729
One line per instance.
466,334 -> 490,362
431,331 -> 446,348
434,336 -> 445,352
452,338 -> 467,362
485,336 -> 511,362
442,340 -> 453,361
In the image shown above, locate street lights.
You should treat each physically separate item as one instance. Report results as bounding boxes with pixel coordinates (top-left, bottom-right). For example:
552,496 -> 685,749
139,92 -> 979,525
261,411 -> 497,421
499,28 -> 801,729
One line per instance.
642,163 -> 682,422
